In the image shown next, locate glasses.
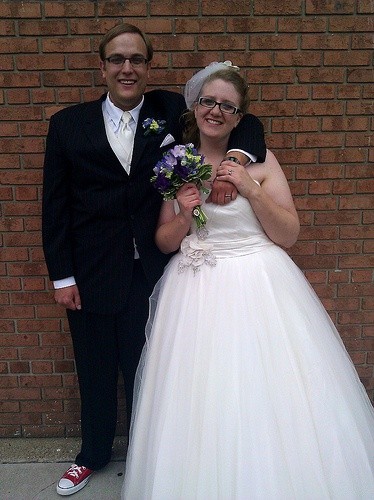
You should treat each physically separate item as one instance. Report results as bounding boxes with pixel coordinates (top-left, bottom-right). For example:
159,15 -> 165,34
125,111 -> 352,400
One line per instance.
199,97 -> 242,115
103,56 -> 148,66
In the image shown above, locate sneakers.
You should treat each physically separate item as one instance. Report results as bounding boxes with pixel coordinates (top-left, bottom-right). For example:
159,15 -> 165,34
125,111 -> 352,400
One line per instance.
57,464 -> 94,495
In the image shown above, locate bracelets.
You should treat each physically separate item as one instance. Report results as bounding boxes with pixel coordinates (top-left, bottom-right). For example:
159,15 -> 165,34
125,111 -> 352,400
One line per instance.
222,157 -> 240,165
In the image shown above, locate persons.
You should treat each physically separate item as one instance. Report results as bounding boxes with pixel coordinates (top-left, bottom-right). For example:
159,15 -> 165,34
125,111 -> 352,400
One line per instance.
121,60 -> 374,500
42,23 -> 267,496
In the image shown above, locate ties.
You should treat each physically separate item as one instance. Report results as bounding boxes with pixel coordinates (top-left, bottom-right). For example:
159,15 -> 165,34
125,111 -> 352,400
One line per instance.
118,111 -> 133,155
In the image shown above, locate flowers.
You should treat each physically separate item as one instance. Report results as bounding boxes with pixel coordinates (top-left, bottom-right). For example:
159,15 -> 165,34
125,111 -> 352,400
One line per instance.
150,143 -> 214,233
141,117 -> 167,136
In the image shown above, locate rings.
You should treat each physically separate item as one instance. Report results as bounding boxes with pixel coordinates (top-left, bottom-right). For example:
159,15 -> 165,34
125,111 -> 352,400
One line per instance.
225,195 -> 231,198
228,170 -> 232,175
